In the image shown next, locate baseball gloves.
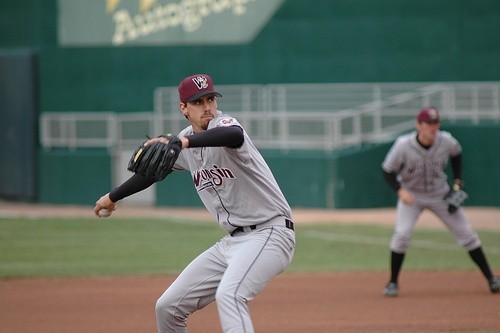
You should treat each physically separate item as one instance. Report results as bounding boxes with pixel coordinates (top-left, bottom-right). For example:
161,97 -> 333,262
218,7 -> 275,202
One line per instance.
127,133 -> 182,181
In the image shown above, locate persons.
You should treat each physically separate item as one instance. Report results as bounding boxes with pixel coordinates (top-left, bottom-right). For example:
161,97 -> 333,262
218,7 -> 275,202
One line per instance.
94,75 -> 295,333
381,106 -> 500,297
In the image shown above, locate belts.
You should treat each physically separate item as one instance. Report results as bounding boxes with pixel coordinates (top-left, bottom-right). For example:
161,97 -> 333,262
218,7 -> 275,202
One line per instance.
232,217 -> 295,235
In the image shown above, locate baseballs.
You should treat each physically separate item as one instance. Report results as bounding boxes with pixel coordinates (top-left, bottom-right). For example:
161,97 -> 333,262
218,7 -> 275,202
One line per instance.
453,184 -> 459,191
99,208 -> 111,217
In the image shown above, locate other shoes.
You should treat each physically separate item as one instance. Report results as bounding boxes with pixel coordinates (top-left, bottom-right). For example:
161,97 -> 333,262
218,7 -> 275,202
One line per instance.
488,274 -> 499,293
383,280 -> 399,296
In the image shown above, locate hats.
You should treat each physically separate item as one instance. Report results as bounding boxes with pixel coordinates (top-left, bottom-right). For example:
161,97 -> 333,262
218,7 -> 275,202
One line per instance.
417,106 -> 442,124
177,74 -> 223,103
